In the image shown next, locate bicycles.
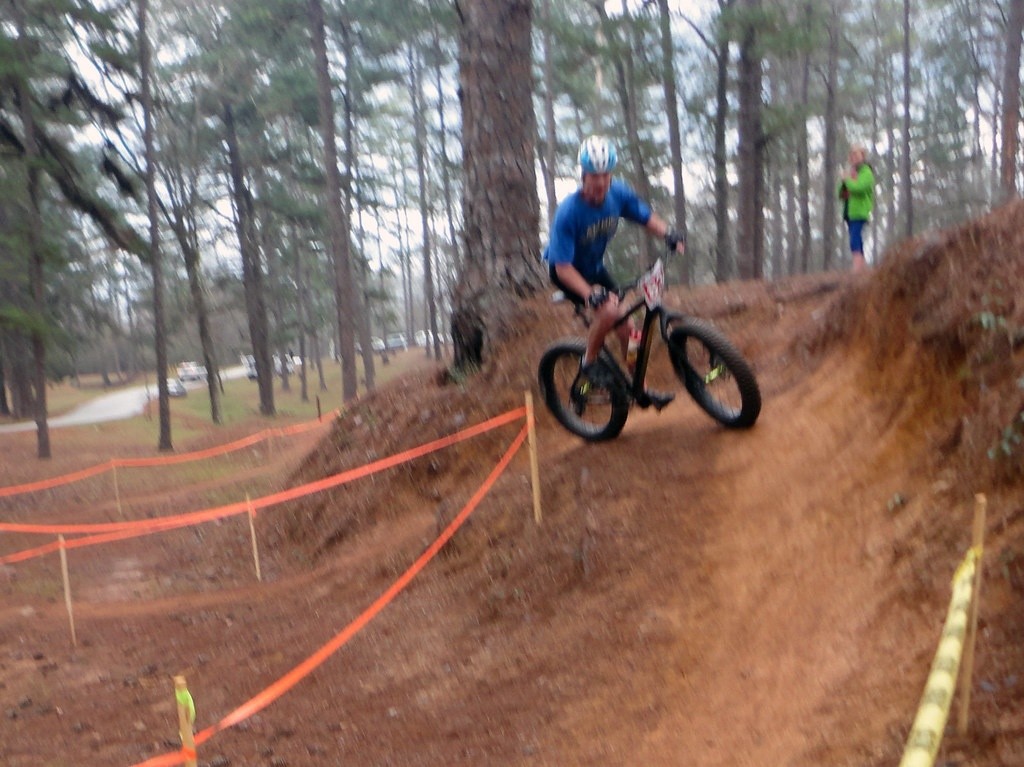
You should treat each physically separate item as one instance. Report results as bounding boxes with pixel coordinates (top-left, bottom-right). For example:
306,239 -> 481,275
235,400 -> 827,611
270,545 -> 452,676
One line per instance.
538,248 -> 763,443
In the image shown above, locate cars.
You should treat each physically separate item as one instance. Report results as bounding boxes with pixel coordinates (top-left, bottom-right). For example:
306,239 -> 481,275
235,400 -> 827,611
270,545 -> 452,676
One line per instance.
245,353 -> 296,380
166,377 -> 186,399
370,329 -> 454,352
176,361 -> 199,382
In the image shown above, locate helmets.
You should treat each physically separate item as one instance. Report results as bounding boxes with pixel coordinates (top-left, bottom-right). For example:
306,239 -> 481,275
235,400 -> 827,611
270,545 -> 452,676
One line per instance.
576,135 -> 619,175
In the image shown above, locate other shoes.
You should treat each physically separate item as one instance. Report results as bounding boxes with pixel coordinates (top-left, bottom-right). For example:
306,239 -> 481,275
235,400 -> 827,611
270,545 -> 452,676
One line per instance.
578,353 -> 614,386
647,388 -> 675,411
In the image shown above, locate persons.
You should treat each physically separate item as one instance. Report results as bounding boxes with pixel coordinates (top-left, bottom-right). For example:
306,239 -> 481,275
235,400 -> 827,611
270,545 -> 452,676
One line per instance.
836,149 -> 878,274
546,133 -> 686,413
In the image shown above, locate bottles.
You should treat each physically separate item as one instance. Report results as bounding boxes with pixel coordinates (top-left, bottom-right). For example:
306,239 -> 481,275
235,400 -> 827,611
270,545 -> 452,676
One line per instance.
626,327 -> 641,366
